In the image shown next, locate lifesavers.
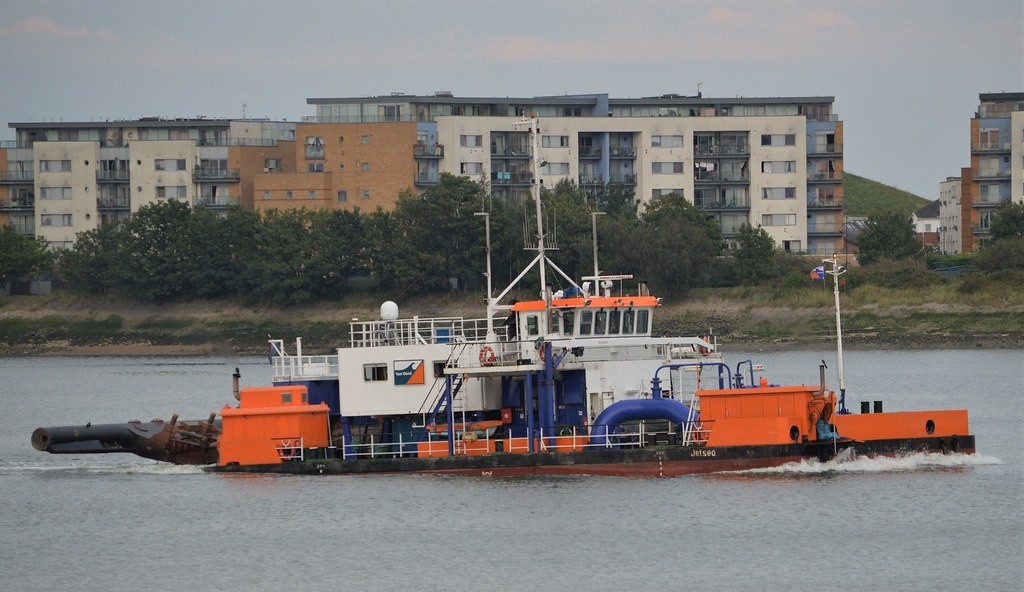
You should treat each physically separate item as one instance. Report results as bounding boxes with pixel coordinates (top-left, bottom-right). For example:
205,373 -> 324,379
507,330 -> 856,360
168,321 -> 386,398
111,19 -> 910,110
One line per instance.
700,337 -> 709,356
279,442 -> 295,461
539,343 -> 546,362
479,346 -> 495,364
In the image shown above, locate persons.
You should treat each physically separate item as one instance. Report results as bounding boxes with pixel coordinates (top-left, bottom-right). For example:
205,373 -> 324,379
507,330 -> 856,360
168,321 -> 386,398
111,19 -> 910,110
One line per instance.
500,298 -> 520,339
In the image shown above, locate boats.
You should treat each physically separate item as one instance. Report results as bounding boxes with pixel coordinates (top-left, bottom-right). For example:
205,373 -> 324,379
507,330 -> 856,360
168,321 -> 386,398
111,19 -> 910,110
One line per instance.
29,115 -> 980,476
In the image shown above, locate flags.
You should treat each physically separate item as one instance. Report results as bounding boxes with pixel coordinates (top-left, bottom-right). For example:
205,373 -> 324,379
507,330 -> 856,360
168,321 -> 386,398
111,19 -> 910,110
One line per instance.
265,333 -> 281,365
809,266 -> 825,281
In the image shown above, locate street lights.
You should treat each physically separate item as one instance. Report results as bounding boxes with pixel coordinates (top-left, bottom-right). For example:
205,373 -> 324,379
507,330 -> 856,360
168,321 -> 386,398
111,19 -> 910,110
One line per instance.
587,212 -> 606,297
472,212 -> 493,299
